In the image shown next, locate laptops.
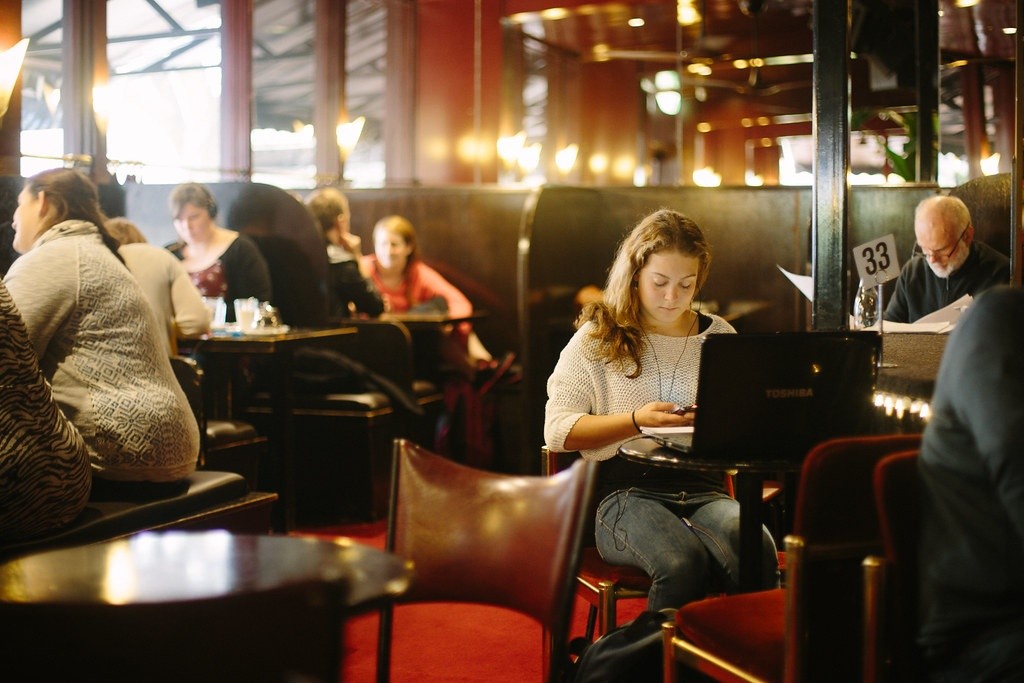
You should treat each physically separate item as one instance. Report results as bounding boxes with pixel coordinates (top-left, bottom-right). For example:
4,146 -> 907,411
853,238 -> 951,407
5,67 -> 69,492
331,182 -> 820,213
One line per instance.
650,330 -> 883,456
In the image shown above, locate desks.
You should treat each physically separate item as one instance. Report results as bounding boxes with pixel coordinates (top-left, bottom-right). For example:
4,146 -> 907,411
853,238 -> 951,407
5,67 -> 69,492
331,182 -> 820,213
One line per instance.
172,320 -> 362,529
877,332 -> 951,429
346,309 -> 487,447
614,436 -> 801,596
0,531 -> 419,683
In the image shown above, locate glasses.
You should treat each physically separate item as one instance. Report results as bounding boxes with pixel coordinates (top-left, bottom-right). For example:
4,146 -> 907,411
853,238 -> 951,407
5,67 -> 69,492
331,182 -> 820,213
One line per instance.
911,223 -> 970,265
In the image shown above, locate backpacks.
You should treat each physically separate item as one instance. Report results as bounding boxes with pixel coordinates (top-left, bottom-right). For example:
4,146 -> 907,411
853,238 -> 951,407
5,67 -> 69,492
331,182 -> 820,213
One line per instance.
557,609 -> 718,683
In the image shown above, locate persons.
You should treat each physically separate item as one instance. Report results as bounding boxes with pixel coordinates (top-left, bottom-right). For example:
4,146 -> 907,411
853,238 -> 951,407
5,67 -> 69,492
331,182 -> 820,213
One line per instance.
362,214 -> 523,397
0,167 -> 199,480
542,211 -> 781,612
884,195 -> 1010,323
912,286 -> 1024,683
105,217 -> 212,357
0,280 -> 92,546
305,188 -> 385,318
159,183 -> 272,323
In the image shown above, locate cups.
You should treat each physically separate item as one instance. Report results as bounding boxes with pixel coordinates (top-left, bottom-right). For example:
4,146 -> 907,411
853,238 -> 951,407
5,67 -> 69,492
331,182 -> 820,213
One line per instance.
234,298 -> 259,330
201,296 -> 227,330
257,307 -> 279,329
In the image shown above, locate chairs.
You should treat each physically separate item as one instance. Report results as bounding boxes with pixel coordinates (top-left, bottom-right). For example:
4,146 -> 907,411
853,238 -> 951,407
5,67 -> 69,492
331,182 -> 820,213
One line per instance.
538,443 -> 652,645
0,579 -> 346,683
170,354 -> 269,490
661,433 -> 924,683
341,437 -> 606,683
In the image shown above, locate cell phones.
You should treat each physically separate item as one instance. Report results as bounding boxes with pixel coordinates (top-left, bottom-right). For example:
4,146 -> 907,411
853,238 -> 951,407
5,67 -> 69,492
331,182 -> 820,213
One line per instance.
673,404 -> 697,415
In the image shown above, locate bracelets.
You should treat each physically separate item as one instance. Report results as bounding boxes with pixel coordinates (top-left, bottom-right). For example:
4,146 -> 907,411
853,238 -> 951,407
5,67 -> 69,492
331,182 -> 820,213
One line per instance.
632,411 -> 642,433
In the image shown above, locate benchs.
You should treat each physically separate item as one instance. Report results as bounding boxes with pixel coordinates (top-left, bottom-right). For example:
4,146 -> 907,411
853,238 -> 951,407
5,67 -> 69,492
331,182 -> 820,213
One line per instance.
0,174 -> 1011,527
0,468 -> 279,576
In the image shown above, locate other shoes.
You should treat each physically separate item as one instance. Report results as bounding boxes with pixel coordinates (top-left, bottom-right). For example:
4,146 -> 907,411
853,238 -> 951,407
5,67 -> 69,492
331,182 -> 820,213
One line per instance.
500,363 -> 524,388
467,352 -> 516,398
